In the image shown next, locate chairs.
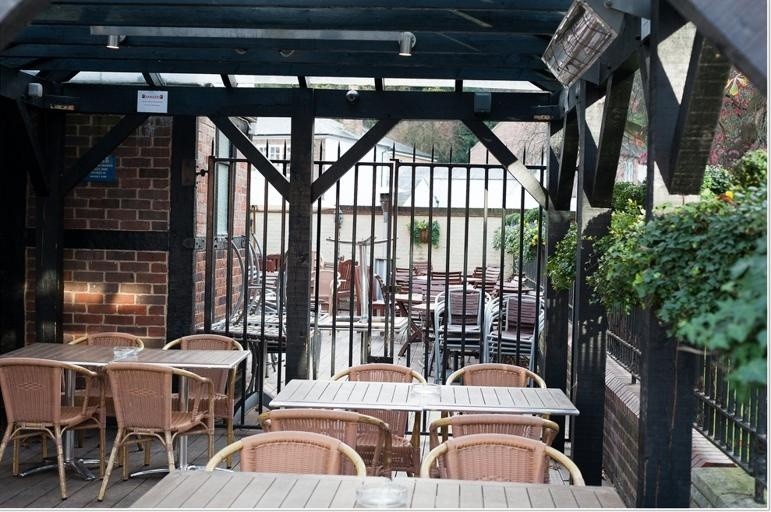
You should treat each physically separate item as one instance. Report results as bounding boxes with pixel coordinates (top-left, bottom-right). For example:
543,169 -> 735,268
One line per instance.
240,232 -> 287,315
331,362 -> 427,478
61,331 -> 144,467
225,234 -> 285,326
434,287 -> 492,385
486,294 -> 546,387
255,408 -> 395,477
205,430 -> 368,478
337,259 -> 359,314
0,357 -> 106,499
429,414 -> 561,477
374,259 -> 535,357
96,362 -> 216,502
162,334 -> 245,469
311,270 -> 342,316
421,433 -> 587,486
441,361 -> 551,444
355,265 -> 399,337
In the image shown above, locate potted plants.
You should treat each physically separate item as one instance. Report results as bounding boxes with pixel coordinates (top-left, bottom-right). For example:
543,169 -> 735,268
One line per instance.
405,218 -> 442,260
